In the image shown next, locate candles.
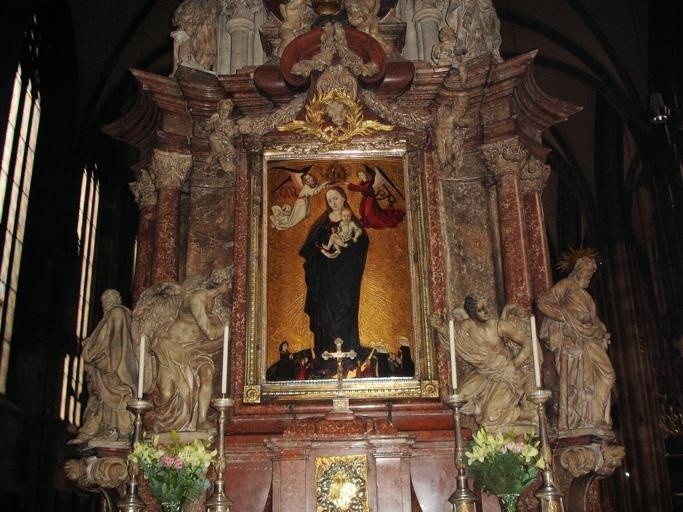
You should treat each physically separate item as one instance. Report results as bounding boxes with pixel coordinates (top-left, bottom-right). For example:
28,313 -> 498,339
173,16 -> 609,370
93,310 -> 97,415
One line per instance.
448,319 -> 458,390
530,316 -> 542,389
136,332 -> 145,396
220,326 -> 228,394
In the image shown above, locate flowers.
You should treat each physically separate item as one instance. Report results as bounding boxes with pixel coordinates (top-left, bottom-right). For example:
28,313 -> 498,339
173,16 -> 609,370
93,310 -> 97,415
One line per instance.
461,426 -> 545,512
126,430 -> 221,512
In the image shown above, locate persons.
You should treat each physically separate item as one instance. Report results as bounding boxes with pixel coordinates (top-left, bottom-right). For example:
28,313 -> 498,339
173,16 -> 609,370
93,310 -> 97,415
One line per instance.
72,288 -> 138,443
173,0 -> 217,68
345,0 -> 381,39
151,270 -> 233,434
428,292 -> 535,432
278,0 -> 311,58
537,250 -> 614,431
444,0 -> 502,61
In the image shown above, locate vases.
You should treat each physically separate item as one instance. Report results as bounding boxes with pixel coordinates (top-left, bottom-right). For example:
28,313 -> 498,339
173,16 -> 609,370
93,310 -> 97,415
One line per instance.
161,502 -> 178,511
496,493 -> 519,512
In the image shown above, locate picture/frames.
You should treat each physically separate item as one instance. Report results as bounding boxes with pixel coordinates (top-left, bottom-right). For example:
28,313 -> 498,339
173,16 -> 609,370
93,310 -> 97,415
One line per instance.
243,136 -> 440,403
